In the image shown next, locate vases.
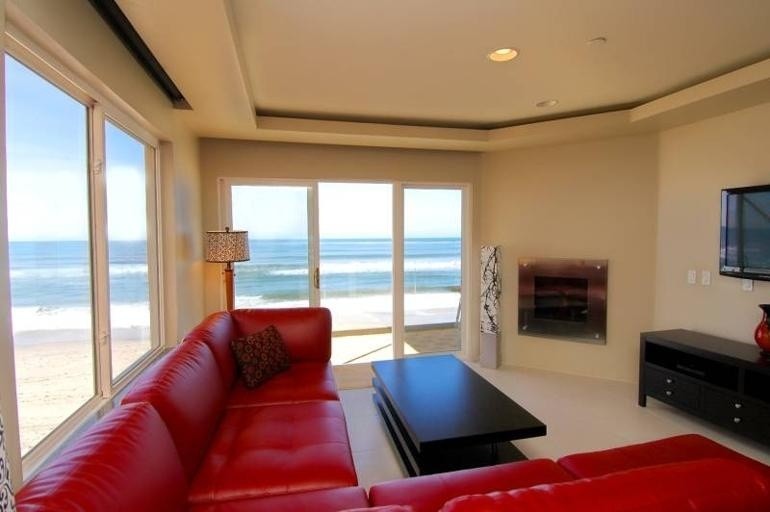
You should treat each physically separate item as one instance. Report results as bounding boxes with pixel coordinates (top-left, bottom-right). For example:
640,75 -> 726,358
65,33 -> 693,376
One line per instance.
754,304 -> 770,356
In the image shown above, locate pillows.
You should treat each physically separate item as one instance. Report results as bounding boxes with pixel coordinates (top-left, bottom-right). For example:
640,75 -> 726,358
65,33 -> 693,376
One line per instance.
232,325 -> 290,388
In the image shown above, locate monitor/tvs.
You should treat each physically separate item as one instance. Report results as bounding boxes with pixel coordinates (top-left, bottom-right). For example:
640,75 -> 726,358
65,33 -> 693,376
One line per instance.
719,184 -> 770,281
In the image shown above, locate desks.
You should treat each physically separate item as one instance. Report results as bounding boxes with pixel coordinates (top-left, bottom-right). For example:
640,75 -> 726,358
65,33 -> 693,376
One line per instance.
638,329 -> 770,447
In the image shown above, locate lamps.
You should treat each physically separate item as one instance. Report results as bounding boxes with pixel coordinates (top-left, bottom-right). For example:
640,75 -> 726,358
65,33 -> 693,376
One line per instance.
205,227 -> 250,310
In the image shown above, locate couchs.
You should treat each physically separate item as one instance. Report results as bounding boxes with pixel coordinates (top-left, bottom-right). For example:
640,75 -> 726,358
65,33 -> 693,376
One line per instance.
14,308 -> 770,512
